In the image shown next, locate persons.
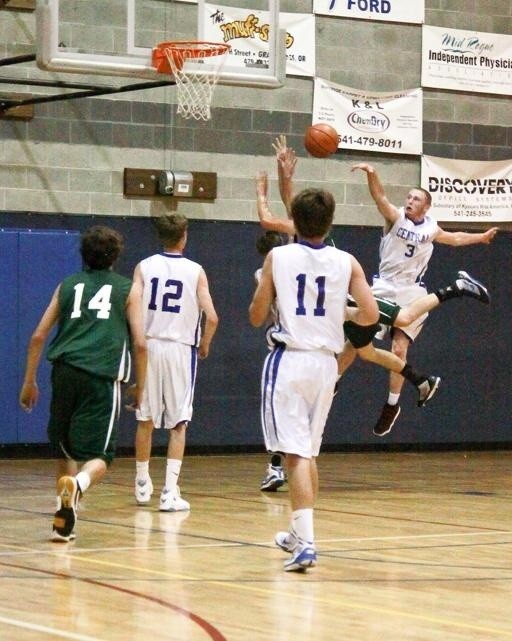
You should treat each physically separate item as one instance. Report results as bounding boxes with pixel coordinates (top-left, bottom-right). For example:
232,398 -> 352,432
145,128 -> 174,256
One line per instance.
248,229 -> 297,490
255,139 -> 490,407
251,192 -> 379,572
131,214 -> 218,512
333,162 -> 498,438
19,223 -> 148,542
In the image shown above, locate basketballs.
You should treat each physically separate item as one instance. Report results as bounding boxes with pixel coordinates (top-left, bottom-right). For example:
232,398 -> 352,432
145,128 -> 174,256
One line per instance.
305,123 -> 339,158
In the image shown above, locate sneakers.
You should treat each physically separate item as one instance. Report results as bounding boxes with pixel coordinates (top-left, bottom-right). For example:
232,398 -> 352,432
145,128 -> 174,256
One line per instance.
275,531 -> 298,552
372,401 -> 401,436
134,476 -> 153,504
449,270 -> 491,306
417,375 -> 441,408
334,382 -> 338,397
50,524 -> 78,542
159,486 -> 190,512
260,462 -> 285,491
283,537 -> 317,571
54,476 -> 83,537
